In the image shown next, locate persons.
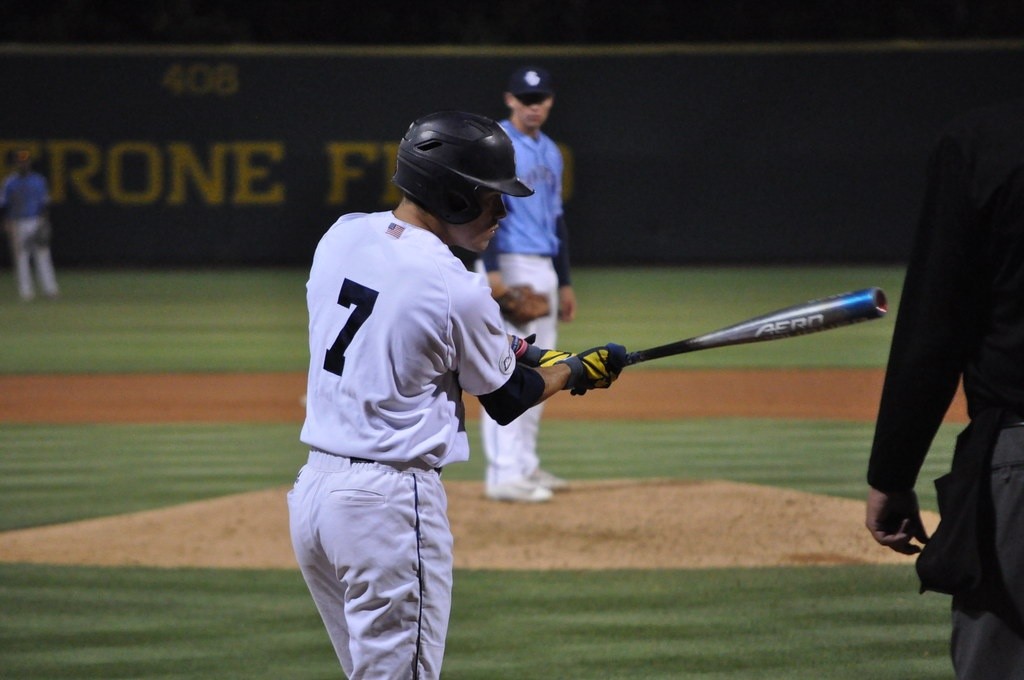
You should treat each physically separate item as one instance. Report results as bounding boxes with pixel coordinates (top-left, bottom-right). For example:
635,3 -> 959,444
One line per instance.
0,149 -> 60,302
287,112 -> 627,680
479,67 -> 576,503
865,95 -> 1024,680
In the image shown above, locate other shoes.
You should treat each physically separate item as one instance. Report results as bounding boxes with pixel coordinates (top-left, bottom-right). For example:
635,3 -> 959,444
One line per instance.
526,469 -> 568,490
485,480 -> 552,503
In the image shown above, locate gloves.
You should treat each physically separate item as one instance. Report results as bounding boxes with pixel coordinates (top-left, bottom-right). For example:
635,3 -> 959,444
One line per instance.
513,333 -> 575,368
555,343 -> 627,395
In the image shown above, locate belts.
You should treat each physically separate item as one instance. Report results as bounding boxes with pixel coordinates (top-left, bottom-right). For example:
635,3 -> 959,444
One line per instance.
351,455 -> 441,474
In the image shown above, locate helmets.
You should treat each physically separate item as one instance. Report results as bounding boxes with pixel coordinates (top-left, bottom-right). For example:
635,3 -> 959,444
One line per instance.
393,111 -> 534,225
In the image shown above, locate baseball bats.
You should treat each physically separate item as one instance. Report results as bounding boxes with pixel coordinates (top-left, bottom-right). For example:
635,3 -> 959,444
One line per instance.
624,284 -> 889,367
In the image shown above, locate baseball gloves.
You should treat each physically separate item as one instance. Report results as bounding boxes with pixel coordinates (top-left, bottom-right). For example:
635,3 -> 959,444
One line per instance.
500,285 -> 553,326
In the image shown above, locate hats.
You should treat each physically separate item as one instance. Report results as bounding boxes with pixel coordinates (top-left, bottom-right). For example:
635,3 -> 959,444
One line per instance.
510,68 -> 554,97
